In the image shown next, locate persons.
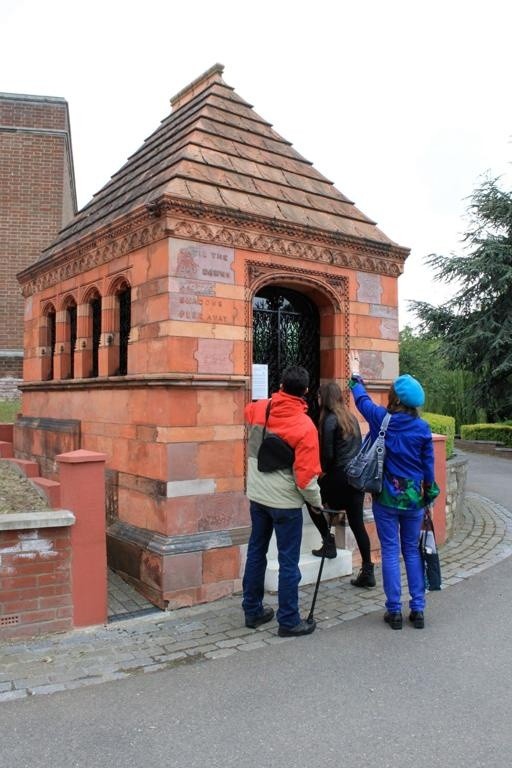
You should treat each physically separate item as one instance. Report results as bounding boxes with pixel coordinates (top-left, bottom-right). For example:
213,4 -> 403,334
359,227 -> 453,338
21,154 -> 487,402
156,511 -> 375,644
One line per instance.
348,374 -> 435,629
306,383 -> 376,587
241,365 -> 324,637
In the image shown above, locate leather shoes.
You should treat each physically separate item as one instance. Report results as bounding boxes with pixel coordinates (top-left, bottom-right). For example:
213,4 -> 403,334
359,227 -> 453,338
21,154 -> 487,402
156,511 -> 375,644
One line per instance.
278,616 -> 317,637
408,608 -> 426,628
384,610 -> 404,630
243,607 -> 274,629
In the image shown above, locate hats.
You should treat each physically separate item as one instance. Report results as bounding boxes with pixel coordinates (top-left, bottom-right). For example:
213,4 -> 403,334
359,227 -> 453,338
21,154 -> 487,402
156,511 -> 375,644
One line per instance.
392,372 -> 426,409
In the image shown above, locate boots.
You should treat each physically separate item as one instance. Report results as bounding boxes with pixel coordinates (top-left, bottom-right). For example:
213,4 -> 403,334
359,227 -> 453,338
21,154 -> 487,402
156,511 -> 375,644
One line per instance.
311,532 -> 338,559
350,560 -> 377,589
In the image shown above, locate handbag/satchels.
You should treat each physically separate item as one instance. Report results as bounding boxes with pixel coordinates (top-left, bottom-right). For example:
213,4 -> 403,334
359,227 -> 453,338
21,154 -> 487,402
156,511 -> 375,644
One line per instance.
419,513 -> 443,593
255,432 -> 294,474
342,411 -> 393,496
